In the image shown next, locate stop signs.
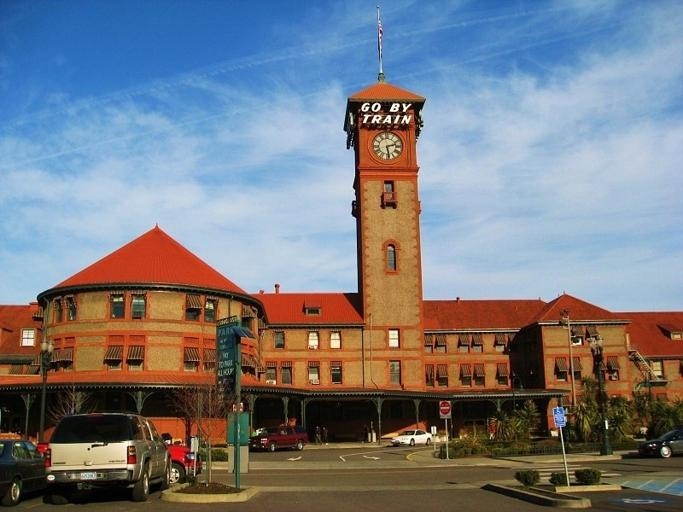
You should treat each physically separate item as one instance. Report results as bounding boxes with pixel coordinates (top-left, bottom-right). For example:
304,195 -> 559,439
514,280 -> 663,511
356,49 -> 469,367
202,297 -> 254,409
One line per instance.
438,400 -> 451,419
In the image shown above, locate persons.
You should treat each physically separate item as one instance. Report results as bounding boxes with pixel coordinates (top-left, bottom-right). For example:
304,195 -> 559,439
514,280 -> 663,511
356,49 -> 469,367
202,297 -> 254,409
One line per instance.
358,423 -> 368,443
314,426 -> 322,445
321,427 -> 328,444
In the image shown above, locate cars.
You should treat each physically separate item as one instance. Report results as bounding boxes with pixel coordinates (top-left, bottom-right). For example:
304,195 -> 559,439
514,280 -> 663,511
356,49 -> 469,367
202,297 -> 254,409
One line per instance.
391,429 -> 432,446
639,429 -> 683,458
0,439 -> 45,507
38,442 -> 202,484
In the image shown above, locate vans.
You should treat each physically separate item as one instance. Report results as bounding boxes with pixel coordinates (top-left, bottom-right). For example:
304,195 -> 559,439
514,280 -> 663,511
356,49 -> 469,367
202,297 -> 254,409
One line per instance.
45,412 -> 172,501
248,426 -> 308,452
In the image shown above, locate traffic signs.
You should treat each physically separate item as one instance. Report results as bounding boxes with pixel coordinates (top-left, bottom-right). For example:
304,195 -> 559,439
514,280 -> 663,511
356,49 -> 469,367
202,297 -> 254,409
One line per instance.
553,406 -> 566,428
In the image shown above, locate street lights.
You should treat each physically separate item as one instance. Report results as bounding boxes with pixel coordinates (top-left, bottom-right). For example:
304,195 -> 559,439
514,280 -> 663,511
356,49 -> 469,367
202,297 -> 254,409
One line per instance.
38,337 -> 54,442
588,335 -> 613,456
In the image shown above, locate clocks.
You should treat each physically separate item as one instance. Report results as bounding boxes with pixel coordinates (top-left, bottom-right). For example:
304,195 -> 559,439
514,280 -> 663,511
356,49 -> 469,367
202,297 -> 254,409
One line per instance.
366,130 -> 406,166
